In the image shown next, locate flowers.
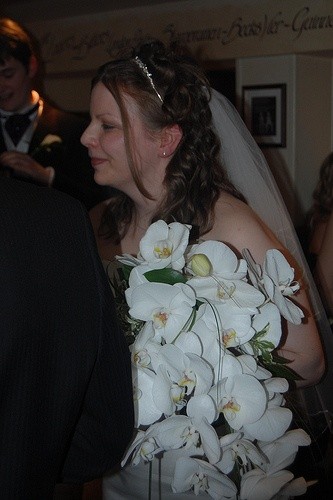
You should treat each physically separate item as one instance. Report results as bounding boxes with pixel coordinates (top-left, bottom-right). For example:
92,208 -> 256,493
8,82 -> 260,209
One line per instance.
108,219 -> 318,500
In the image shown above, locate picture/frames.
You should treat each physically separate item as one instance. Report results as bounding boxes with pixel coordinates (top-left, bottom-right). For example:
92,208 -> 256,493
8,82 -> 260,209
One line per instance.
239,83 -> 288,148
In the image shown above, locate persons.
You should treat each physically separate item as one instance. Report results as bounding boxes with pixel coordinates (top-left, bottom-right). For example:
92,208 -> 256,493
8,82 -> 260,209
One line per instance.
0,17 -> 333,500
